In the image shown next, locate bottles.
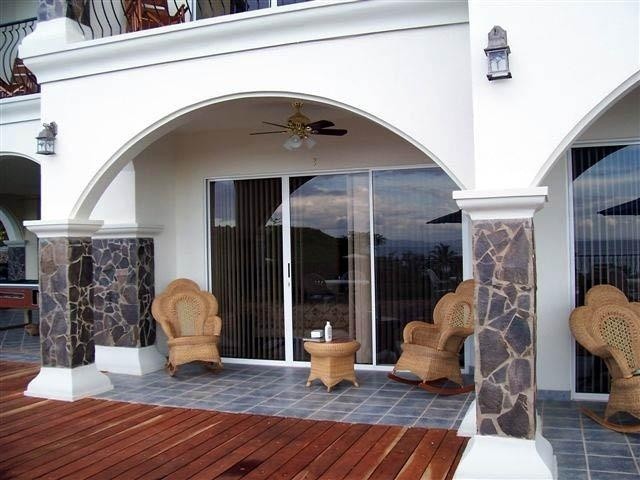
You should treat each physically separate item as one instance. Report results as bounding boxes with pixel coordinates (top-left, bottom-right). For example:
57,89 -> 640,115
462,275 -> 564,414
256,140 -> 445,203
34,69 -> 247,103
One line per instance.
324,321 -> 333,343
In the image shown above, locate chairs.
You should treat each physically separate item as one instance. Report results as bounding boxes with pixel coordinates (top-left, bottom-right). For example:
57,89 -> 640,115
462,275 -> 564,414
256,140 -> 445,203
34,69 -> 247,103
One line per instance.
119,0 -> 191,33
0,56 -> 41,94
385,276 -> 476,397
567,280 -> 639,435
150,278 -> 226,378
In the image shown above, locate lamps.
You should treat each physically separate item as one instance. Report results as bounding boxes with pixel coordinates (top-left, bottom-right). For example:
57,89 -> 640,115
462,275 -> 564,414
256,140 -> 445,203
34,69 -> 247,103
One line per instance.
36,121 -> 58,156
282,136 -> 318,150
483,23 -> 514,80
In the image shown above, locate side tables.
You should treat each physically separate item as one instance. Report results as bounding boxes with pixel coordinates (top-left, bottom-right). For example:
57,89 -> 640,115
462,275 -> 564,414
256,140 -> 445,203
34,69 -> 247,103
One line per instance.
303,337 -> 361,394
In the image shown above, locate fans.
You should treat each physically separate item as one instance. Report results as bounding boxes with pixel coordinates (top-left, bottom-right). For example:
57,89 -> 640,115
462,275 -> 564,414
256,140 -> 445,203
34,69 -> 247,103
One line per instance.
249,99 -> 348,137
